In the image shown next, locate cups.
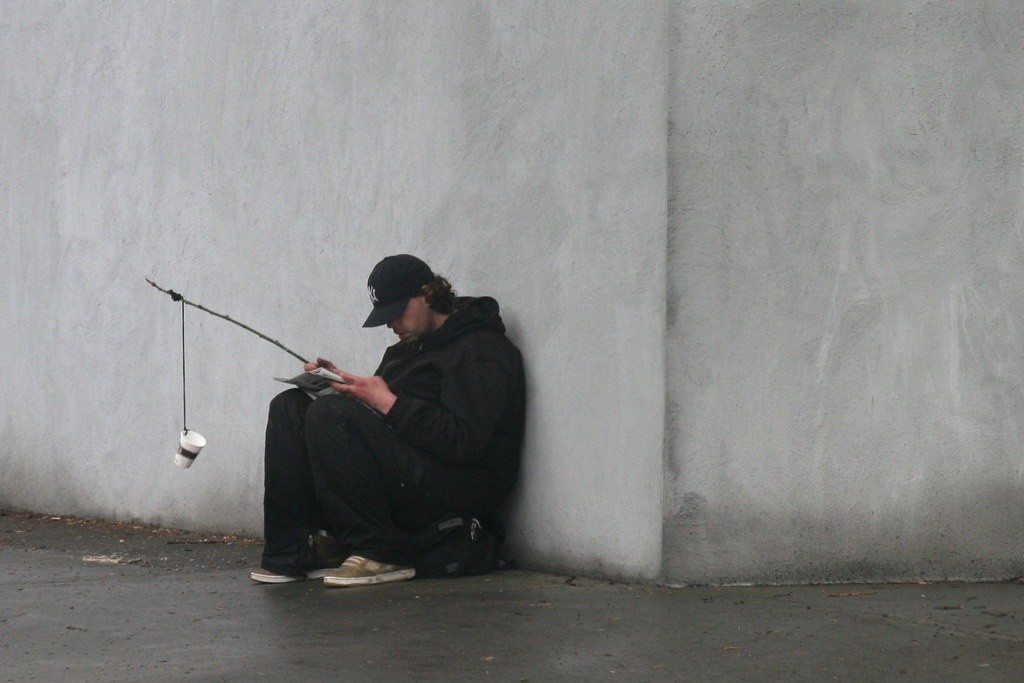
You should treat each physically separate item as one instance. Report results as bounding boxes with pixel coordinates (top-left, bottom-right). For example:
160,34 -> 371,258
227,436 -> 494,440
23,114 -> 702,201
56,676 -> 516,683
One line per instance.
175,431 -> 206,468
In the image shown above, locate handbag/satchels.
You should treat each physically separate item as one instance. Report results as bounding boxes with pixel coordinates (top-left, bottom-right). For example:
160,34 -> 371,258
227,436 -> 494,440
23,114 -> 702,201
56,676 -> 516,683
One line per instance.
413,509 -> 507,577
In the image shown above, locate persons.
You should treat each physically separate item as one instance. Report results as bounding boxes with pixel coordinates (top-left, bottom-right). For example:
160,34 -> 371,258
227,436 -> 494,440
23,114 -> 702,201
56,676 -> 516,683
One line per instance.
250,255 -> 527,584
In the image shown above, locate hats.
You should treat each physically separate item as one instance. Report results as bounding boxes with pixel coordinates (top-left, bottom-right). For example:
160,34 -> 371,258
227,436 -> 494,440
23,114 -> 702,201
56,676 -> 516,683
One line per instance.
361,254 -> 435,327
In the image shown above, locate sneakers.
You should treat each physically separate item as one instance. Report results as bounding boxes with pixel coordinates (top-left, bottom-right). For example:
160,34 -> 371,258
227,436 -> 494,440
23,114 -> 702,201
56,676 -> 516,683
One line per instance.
249,565 -> 340,583
322,555 -> 417,586
